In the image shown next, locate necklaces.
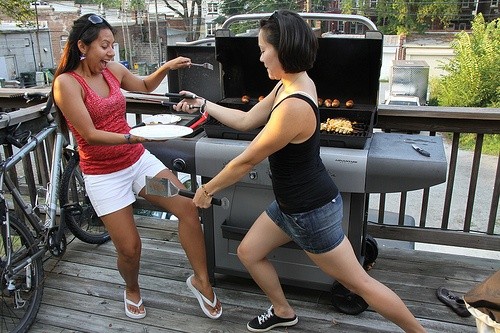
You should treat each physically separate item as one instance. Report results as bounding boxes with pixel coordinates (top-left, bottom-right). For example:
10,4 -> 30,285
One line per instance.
278,77 -> 298,95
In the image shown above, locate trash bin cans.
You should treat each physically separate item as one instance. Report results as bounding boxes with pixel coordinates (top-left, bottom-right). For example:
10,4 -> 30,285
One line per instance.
41,68 -> 54,83
0,79 -> 5,88
148,64 -> 158,75
138,62 -> 147,76
120,61 -> 128,68
21,72 -> 36,88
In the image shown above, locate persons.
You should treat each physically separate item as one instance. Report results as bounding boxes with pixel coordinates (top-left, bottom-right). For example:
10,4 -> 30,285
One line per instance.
437,269 -> 500,333
51,13 -> 222,320
173,8 -> 426,333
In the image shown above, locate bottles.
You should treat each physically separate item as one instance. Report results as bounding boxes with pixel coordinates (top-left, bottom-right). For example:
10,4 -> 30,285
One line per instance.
37,183 -> 47,214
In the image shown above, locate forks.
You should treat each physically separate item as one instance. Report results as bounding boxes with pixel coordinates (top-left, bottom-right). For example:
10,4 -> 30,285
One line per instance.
189,62 -> 213,70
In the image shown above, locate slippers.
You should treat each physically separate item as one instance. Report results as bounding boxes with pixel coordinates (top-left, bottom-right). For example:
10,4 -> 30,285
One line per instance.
124,290 -> 146,319
186,274 -> 222,319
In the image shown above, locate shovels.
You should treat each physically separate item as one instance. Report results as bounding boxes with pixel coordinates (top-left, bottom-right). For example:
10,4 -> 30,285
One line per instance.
145,176 -> 222,206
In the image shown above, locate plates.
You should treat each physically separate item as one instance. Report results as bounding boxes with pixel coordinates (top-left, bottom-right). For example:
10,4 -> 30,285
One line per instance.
129,124 -> 194,141
142,114 -> 182,123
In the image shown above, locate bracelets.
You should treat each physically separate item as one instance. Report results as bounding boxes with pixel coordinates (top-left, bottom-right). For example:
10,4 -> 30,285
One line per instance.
125,134 -> 132,144
201,184 -> 214,198
200,99 -> 207,114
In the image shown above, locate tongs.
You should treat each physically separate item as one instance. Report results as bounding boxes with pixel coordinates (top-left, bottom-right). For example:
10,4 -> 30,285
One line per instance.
126,89 -> 206,109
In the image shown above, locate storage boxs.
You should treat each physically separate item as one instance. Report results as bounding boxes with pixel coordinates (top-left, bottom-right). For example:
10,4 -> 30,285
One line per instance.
388,59 -> 430,101
20,72 -> 44,86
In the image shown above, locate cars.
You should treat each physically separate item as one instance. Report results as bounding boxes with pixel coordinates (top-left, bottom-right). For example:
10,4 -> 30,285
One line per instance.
382,95 -> 426,134
391,73 -> 417,96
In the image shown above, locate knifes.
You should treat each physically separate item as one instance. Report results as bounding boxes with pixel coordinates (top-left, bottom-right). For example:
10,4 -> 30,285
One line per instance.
412,143 -> 430,156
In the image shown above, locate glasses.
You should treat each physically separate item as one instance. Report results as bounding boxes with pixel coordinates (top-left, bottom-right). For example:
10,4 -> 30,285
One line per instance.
268,10 -> 280,26
81,15 -> 107,34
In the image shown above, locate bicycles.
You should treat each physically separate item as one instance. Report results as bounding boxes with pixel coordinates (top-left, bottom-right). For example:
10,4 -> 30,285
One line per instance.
0,91 -> 111,333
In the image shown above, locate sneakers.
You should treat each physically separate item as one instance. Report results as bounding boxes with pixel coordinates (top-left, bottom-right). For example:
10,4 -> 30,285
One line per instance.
246,304 -> 298,332
437,286 -> 472,318
